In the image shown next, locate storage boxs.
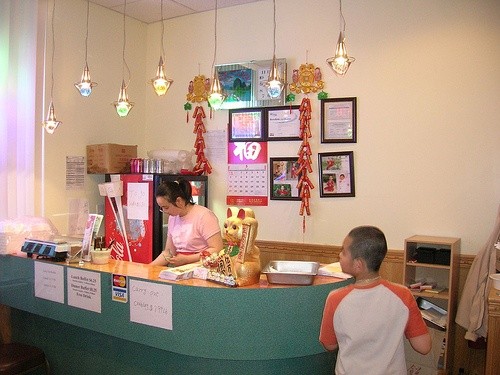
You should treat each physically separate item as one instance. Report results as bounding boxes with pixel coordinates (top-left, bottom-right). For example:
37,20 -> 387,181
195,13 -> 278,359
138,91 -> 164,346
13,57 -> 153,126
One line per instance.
87,143 -> 137,173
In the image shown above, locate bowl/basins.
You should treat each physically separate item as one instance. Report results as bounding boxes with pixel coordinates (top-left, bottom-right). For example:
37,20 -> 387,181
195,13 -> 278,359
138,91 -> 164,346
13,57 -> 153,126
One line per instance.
489,274 -> 500,290
261,260 -> 318,285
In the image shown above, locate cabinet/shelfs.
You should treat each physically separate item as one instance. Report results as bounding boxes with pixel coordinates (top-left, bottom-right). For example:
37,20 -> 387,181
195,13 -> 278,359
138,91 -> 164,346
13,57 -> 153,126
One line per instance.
404,236 -> 461,375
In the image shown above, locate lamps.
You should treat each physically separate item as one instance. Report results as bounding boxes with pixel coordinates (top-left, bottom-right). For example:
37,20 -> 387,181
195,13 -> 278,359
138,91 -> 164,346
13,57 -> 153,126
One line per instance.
327,0 -> 355,76
74,0 -> 98,98
208,0 -> 226,110
266,0 -> 284,97
152,0 -> 173,95
42,0 -> 61,134
112,0 -> 136,117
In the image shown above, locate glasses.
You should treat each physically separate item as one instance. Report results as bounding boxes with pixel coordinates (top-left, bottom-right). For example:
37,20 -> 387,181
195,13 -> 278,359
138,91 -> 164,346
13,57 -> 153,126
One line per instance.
159,206 -> 168,214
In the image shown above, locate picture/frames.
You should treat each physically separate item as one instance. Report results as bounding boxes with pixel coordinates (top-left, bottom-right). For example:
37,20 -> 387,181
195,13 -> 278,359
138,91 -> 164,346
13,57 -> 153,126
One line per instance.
271,156 -> 302,201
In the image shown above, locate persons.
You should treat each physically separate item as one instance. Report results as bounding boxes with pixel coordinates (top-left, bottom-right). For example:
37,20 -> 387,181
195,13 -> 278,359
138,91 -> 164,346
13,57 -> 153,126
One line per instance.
324,174 -> 336,192
326,156 -> 334,171
317,226 -> 433,375
148,177 -> 224,266
273,165 -> 285,180
337,173 -> 350,192
289,162 -> 298,179
275,184 -> 290,196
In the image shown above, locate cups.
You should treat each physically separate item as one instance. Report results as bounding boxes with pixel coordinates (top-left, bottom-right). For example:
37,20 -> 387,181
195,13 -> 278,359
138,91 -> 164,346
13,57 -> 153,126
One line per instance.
149,151 -> 188,171
91,248 -> 112,265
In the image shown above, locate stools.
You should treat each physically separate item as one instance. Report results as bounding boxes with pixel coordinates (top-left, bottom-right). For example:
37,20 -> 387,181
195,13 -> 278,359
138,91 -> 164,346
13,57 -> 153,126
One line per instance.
0,342 -> 52,375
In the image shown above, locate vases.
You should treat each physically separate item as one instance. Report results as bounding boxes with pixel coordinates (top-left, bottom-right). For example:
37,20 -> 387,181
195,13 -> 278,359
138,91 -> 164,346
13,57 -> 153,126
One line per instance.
319,152 -> 356,198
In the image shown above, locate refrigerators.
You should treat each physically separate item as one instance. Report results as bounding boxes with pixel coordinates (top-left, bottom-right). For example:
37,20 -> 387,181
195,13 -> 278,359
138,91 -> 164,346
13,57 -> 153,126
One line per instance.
104,173 -> 210,267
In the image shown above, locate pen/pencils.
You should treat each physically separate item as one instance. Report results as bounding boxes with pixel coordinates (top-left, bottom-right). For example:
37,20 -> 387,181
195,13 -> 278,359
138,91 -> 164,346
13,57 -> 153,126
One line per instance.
94,234 -> 115,251
